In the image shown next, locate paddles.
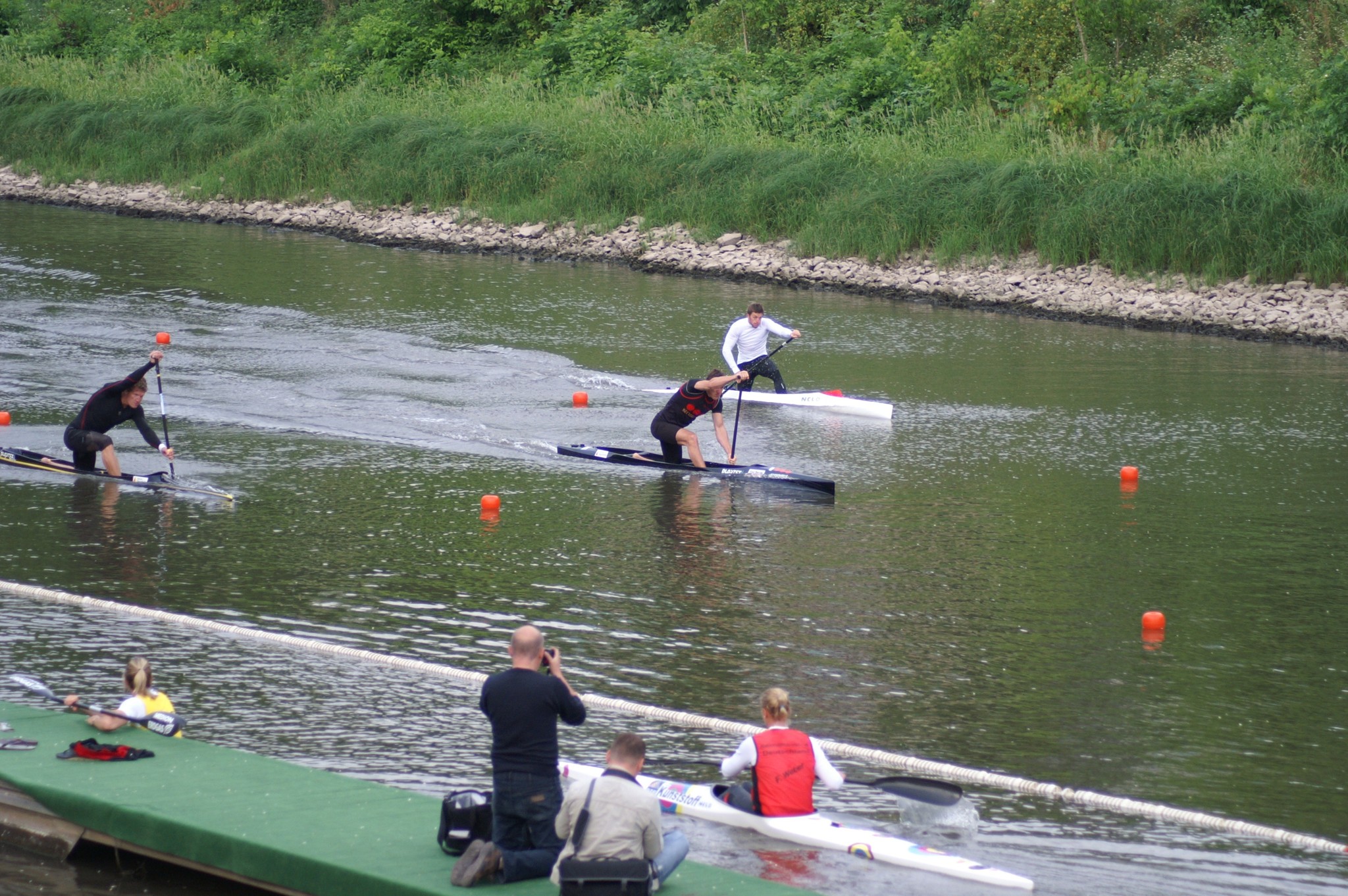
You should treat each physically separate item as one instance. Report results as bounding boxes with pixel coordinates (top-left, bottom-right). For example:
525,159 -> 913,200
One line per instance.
7,675 -> 188,738
150,354 -> 176,478
643,757 -> 964,807
731,375 -> 745,459
724,337 -> 794,389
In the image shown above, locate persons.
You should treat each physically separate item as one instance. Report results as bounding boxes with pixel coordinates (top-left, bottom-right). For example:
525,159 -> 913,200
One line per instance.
722,303 -> 801,394
548,733 -> 690,893
40,350 -> 175,477
450,624 -> 586,887
65,656 -> 182,739
651,368 -> 749,468
714,686 -> 849,818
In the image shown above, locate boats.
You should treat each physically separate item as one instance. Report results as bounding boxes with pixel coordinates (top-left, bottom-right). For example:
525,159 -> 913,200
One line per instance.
0,446 -> 234,502
555,757 -> 1038,893
557,440 -> 836,495
643,381 -> 893,419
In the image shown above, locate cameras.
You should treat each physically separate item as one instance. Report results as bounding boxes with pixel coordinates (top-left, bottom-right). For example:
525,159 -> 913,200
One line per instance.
541,649 -> 554,667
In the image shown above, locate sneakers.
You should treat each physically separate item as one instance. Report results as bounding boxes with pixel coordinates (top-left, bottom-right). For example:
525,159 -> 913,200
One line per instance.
462,841 -> 503,888
450,839 -> 485,885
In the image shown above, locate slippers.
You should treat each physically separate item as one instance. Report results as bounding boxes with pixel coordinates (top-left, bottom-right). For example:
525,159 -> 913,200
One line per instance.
0,736 -> 38,750
0,722 -> 14,732
56,748 -> 99,761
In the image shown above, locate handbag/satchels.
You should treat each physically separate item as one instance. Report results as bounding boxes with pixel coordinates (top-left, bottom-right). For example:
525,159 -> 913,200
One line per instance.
558,856 -> 650,896
437,788 -> 493,857
